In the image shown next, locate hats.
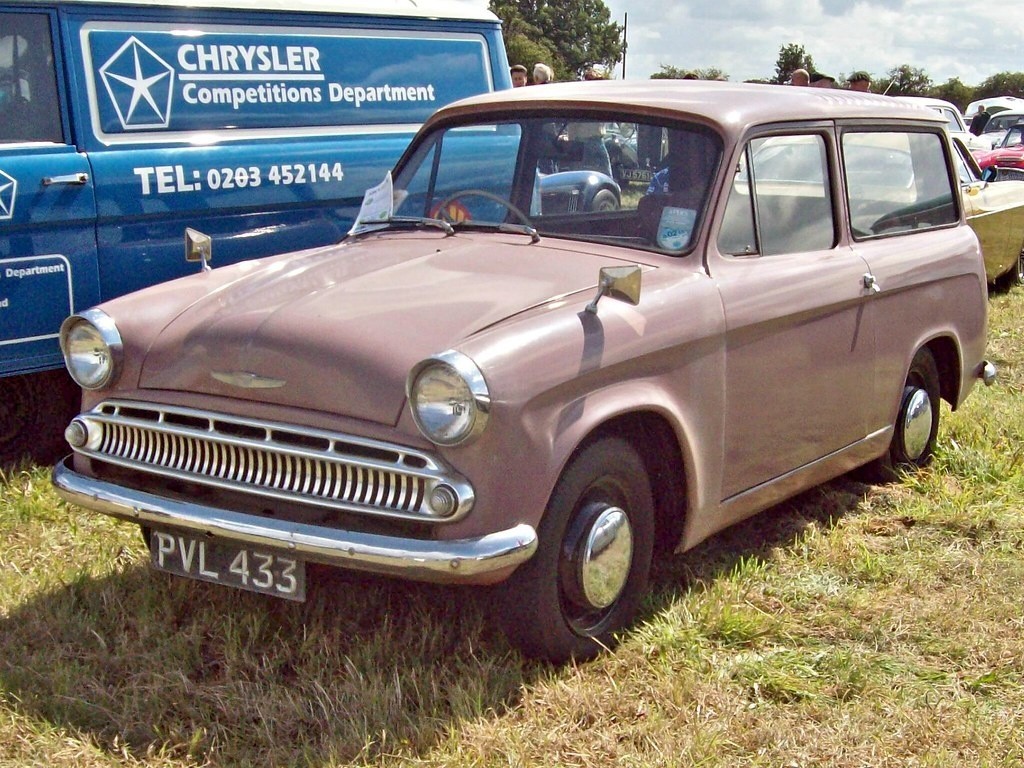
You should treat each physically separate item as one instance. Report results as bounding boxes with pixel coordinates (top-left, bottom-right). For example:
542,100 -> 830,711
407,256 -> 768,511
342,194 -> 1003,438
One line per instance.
847,71 -> 871,82
811,72 -> 835,82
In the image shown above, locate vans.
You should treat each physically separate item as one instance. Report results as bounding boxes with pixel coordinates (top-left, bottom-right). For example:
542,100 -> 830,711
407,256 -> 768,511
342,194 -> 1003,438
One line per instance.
0,0 -> 628,469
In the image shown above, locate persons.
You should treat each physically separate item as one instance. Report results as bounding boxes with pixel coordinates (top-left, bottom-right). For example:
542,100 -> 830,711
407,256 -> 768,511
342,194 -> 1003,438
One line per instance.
788,69 -> 873,93
636,73 -> 699,198
504,62 -> 615,228
968,104 -> 992,137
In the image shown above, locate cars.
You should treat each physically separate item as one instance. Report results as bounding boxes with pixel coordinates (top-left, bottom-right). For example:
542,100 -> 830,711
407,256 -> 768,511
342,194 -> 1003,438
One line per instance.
718,92 -> 1024,296
42,76 -> 998,667
